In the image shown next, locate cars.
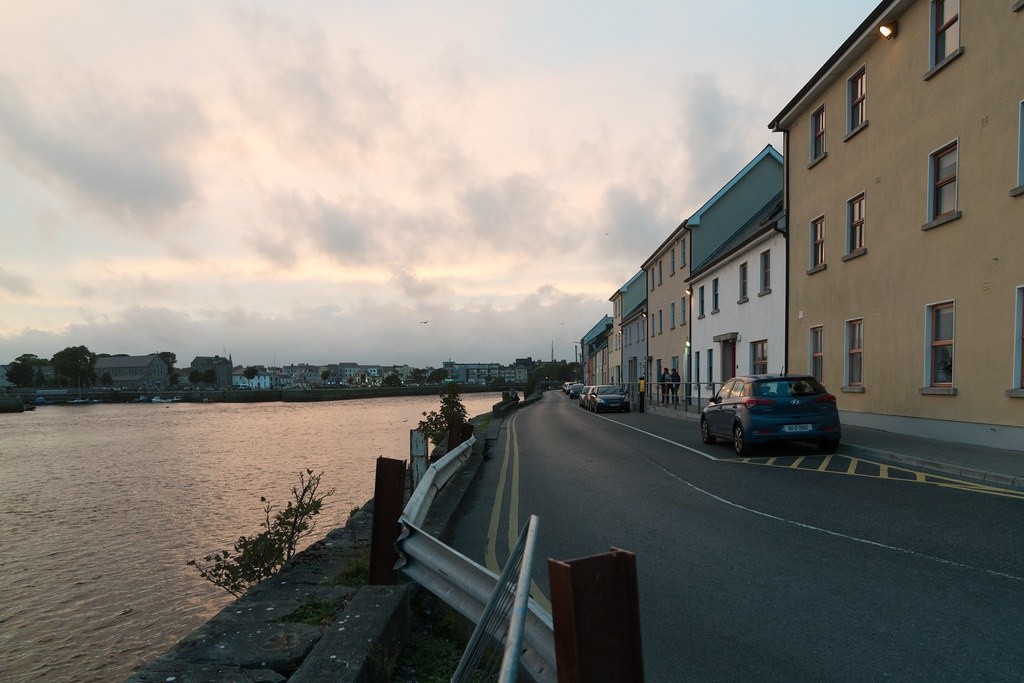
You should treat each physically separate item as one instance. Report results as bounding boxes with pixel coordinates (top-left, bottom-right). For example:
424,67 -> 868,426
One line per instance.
587,385 -> 630,414
562,382 -> 576,394
579,386 -> 591,408
701,373 -> 841,456
569,384 -> 584,399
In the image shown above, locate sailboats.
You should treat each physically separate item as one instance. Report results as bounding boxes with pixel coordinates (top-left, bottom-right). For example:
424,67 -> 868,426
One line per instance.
66,375 -> 89,406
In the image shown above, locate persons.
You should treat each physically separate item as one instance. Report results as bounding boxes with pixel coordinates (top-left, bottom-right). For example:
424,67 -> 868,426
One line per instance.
660,368 -> 681,404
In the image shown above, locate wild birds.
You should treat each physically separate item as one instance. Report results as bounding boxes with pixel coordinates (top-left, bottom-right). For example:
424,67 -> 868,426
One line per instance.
418,320 -> 431,324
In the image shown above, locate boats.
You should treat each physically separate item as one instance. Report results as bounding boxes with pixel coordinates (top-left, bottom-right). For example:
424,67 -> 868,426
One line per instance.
152,395 -> 184,403
29,397 -> 56,406
